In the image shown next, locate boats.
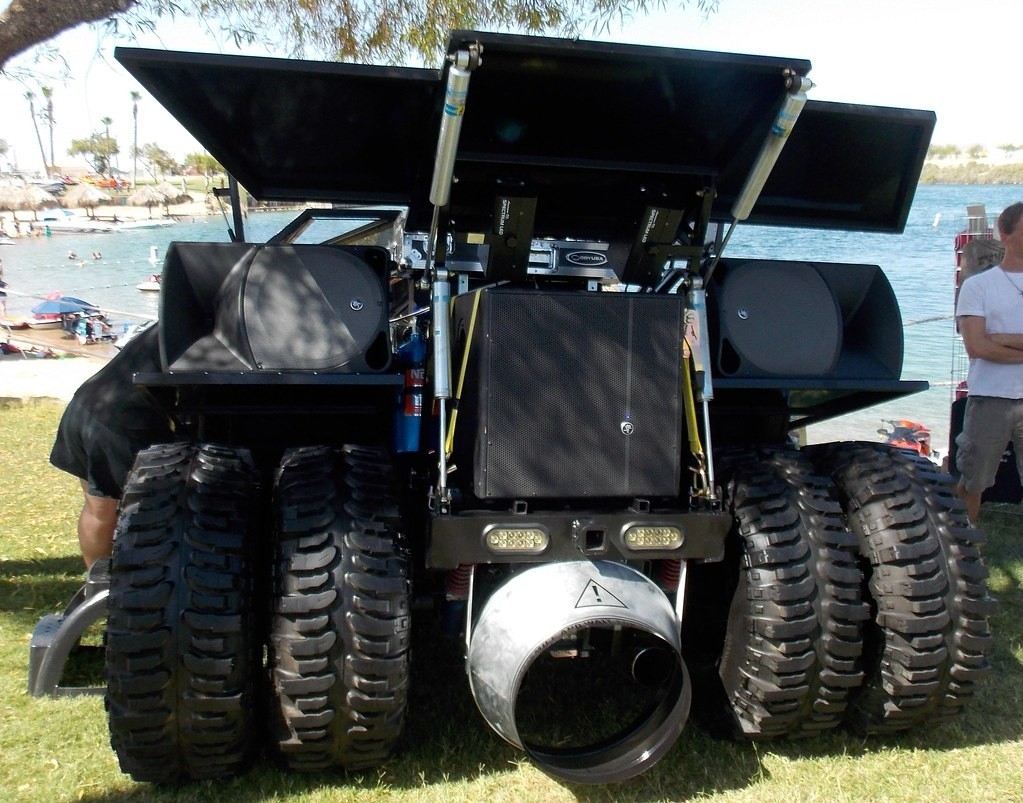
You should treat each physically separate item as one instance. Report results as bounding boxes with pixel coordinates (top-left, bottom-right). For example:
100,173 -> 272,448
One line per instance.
136,273 -> 162,292
0,312 -> 64,331
32,215 -> 180,235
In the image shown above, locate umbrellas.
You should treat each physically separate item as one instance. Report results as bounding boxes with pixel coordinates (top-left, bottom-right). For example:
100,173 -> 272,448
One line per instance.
0,184 -> 57,225
62,183 -> 112,217
128,186 -> 170,219
157,182 -> 192,215
33,297 -> 95,313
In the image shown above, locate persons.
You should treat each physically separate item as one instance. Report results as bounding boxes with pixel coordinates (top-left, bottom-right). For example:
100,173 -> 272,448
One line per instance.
110,176 -> 122,192
955,202 -> 1023,523
71,314 -> 110,336
49,323 -> 165,573
12,218 -> 51,237
0,259 -> 9,317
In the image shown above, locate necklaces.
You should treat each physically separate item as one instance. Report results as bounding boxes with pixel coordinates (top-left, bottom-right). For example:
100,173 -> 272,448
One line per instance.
998,264 -> 1023,297
